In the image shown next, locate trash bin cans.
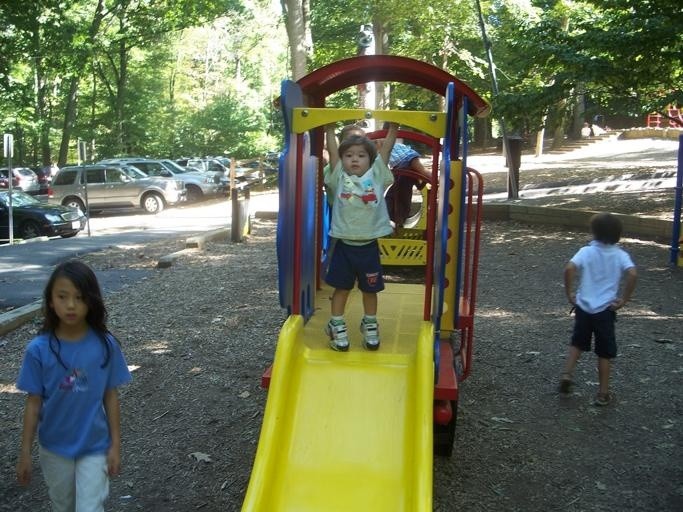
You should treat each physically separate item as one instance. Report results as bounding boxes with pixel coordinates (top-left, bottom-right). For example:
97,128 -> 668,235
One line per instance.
232,188 -> 251,242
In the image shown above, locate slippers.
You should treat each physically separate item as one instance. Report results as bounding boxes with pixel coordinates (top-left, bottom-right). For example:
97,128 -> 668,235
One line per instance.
595,392 -> 612,406
559,374 -> 571,398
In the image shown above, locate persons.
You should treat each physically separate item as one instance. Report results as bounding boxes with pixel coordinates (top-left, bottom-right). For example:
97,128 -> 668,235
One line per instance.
15,262 -> 134,512
558,213 -> 637,406
315,118 -> 433,352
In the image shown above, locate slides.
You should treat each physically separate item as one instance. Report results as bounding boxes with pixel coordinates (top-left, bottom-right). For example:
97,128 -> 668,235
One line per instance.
238,283 -> 434,511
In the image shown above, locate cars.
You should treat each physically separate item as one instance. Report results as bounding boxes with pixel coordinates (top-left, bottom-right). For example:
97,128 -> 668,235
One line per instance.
0,158 -> 264,240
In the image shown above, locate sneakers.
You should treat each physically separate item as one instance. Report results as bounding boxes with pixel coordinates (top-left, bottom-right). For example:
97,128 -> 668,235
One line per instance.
360,319 -> 380,350
324,320 -> 350,352
